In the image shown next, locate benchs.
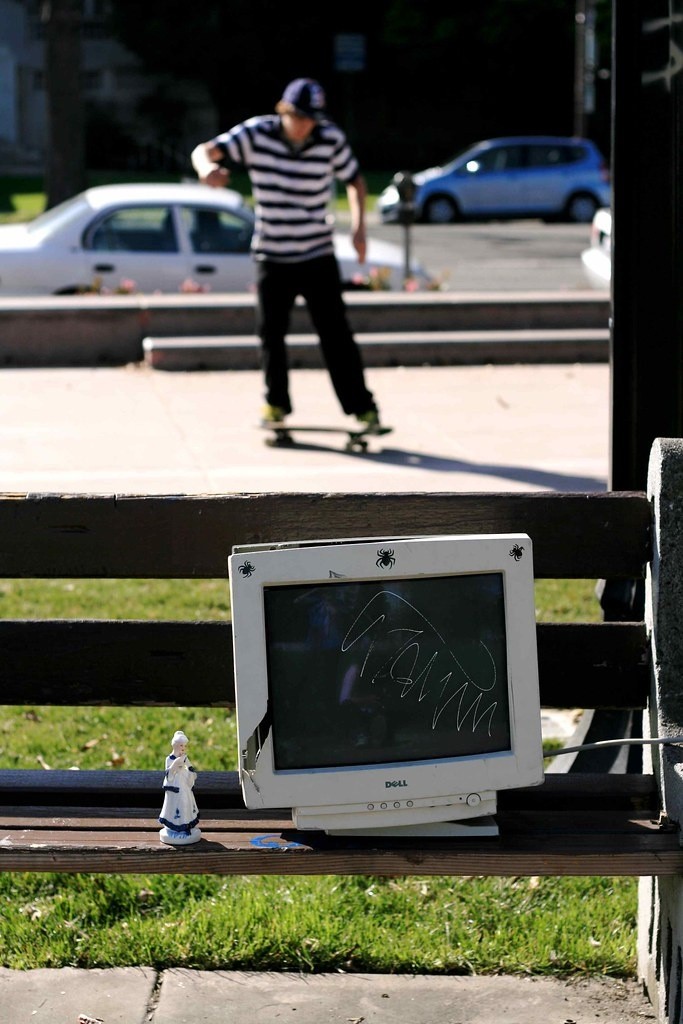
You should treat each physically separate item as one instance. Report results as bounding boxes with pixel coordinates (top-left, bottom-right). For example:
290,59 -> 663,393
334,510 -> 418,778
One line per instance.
0,437 -> 683,1024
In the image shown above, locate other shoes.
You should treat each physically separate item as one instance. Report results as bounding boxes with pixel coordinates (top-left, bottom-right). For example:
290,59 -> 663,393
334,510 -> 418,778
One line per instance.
346,411 -> 390,434
258,405 -> 284,423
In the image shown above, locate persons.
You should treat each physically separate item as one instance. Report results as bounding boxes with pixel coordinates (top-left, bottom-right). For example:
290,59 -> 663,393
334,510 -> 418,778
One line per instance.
158,730 -> 201,836
191,78 -> 381,430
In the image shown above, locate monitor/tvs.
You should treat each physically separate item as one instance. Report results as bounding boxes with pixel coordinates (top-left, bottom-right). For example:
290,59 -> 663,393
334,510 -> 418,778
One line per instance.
226,532 -> 545,839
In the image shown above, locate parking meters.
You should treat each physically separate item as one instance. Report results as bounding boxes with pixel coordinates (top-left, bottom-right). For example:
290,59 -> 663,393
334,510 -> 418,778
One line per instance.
395,174 -> 429,293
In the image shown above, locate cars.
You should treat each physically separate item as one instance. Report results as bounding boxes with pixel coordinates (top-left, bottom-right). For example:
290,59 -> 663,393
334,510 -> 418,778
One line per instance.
378,133 -> 612,227
0,180 -> 436,294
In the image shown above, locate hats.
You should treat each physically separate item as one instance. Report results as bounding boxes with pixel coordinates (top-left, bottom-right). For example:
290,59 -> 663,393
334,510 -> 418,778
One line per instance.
282,79 -> 330,126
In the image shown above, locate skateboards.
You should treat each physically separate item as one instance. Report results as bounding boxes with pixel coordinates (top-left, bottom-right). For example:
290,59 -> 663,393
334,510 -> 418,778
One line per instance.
259,417 -> 393,454
